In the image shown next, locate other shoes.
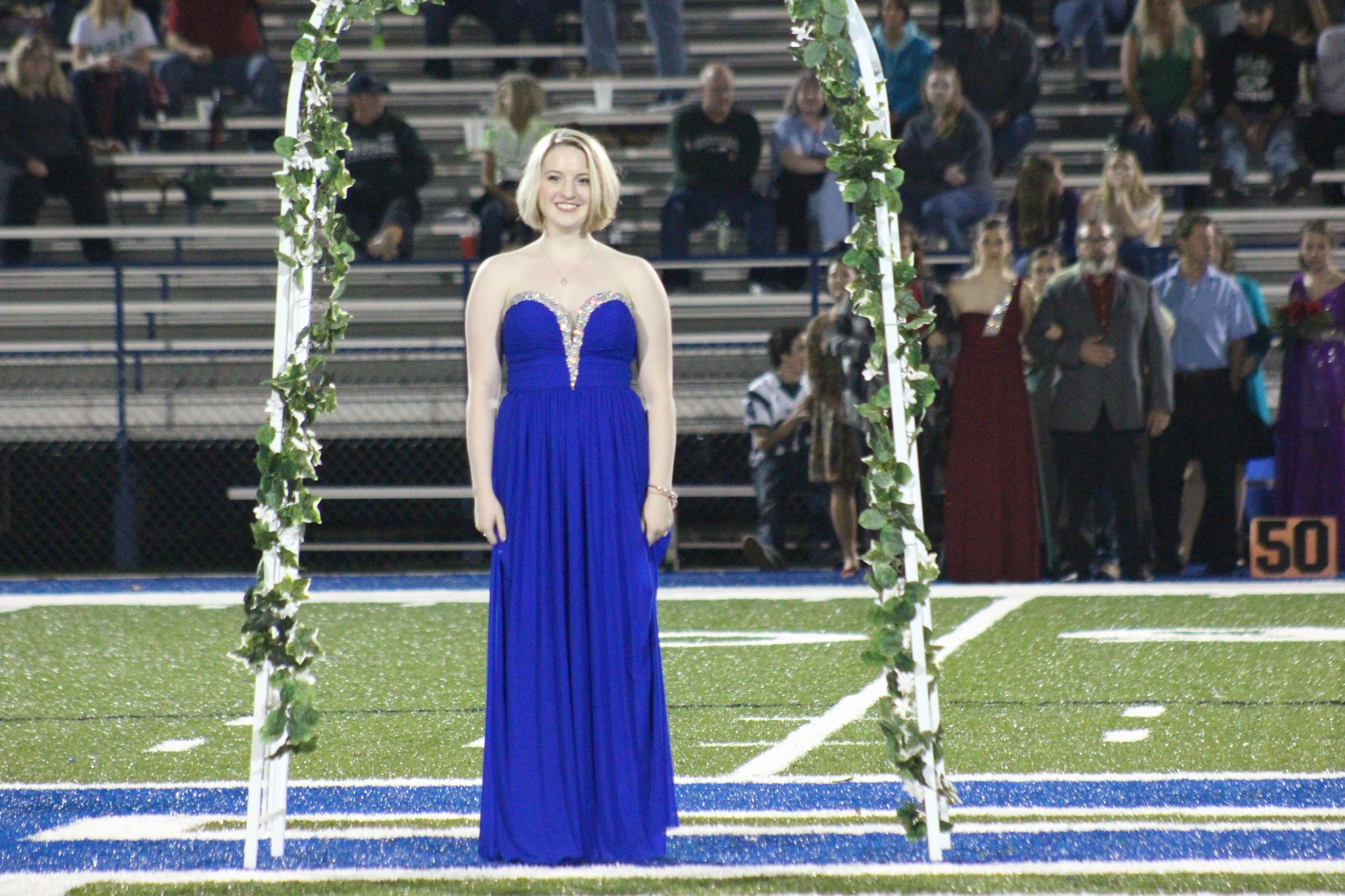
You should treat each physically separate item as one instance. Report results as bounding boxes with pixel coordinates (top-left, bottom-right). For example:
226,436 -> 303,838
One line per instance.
838,564 -> 857,580
367,226 -> 405,262
743,537 -> 781,573
1129,561 -> 1154,582
1091,562 -> 1121,580
1271,166 -> 1313,207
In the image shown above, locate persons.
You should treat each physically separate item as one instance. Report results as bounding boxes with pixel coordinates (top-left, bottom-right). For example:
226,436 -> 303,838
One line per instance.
1000,151 -> 1081,280
745,206 -> 1268,611
761,73 -> 870,275
857,0 -> 1345,201
1076,149 -> 1169,282
66,1 -> 158,158
0,1 -> 61,57
0,31 -> 118,267
464,127 -> 682,863
321,66 -> 435,265
1267,217 -> 1345,568
465,72 -> 560,273
894,61 -> 997,284
654,61 -> 782,299
159,0 -> 284,152
413,0 -> 689,104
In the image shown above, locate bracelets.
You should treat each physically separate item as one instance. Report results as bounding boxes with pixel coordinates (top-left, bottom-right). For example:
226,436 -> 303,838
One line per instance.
646,483 -> 678,510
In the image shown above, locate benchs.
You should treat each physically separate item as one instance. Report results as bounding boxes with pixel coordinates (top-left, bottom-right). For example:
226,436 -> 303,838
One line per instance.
7,0 -> 1342,402
230,484 -> 762,570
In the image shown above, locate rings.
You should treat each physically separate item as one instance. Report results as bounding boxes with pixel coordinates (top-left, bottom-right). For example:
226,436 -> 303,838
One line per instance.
660,532 -> 669,537
482,533 -> 487,537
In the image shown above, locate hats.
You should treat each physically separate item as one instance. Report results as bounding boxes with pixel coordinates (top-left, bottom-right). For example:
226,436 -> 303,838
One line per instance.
965,0 -> 998,30
346,75 -> 392,96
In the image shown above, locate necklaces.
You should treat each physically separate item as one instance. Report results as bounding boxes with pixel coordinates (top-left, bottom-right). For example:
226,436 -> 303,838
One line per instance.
535,233 -> 597,285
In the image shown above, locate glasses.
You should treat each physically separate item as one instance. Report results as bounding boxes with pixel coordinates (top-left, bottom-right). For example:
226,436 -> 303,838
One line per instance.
1077,236 -> 1111,247
